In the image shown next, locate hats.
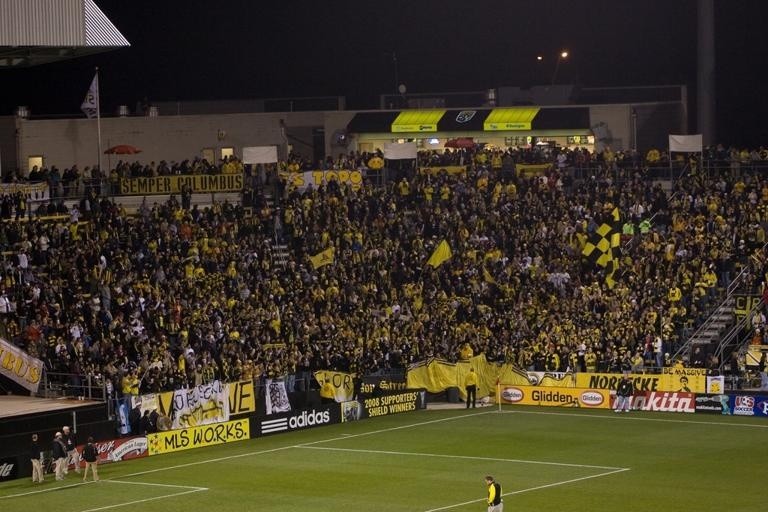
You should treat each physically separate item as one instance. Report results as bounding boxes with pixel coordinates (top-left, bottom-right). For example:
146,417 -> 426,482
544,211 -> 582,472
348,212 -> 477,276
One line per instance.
63,426 -> 69,430
55,432 -> 62,437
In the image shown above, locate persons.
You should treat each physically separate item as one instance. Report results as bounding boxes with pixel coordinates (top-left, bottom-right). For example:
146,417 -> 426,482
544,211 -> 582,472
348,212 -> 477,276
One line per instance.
60,425 -> 81,475
1,138 -> 767,433
51,431 -> 66,481
484,475 -> 504,512
28,433 -> 45,484
81,436 -> 100,481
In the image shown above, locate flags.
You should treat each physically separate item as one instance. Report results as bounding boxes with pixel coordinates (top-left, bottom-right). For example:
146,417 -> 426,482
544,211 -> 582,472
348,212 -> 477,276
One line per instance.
80,73 -> 100,121
